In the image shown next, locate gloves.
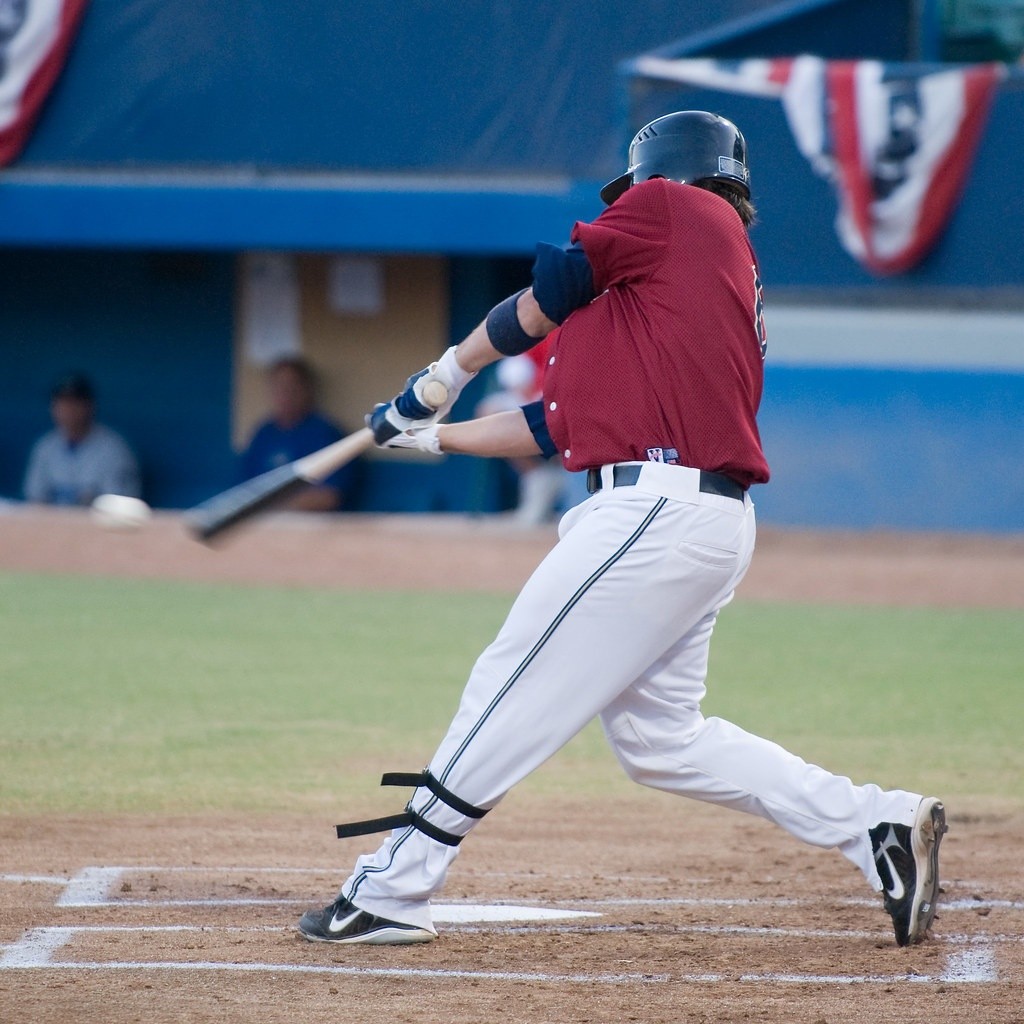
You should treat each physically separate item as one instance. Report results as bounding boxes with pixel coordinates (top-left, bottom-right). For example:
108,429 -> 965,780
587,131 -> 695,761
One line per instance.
393,346 -> 480,429
363,403 -> 447,455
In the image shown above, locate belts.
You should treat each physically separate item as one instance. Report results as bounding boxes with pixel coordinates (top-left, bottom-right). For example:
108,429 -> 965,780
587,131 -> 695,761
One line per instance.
586,465 -> 744,501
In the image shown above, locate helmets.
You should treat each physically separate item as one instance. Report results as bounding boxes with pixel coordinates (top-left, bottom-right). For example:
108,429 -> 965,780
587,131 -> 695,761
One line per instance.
600,110 -> 750,190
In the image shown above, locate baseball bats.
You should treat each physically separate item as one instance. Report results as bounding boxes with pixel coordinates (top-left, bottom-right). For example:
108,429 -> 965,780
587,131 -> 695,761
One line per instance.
187,381 -> 446,543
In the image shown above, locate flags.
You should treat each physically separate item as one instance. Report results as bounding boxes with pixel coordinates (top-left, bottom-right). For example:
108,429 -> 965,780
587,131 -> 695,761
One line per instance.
0,0 -> 87,168
636,53 -> 1002,275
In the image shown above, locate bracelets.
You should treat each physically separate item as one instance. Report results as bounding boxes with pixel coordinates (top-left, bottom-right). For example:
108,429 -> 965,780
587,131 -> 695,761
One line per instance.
521,399 -> 559,459
485,287 -> 548,356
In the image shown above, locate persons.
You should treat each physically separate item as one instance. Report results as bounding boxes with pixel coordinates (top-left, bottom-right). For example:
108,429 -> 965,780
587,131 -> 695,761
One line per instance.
24,374 -> 142,509
474,354 -> 566,524
294,110 -> 947,947
225,355 -> 352,515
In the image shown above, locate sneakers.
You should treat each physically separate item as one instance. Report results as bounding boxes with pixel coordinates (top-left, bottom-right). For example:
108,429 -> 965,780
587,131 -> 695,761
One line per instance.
874,796 -> 948,946
297,892 -> 433,945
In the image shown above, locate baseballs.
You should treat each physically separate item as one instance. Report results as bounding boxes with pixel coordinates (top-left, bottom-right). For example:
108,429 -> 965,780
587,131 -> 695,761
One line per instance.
96,494 -> 147,530
